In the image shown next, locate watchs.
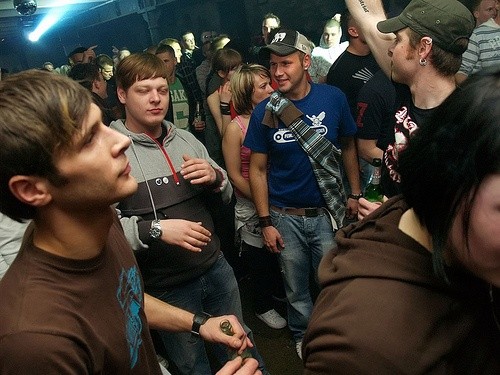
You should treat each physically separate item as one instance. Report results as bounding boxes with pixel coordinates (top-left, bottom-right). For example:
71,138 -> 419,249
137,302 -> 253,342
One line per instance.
149,220 -> 162,242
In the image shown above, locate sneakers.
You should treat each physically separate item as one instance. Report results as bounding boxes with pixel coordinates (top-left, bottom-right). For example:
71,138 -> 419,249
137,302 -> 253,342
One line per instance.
294,340 -> 303,359
255,308 -> 287,329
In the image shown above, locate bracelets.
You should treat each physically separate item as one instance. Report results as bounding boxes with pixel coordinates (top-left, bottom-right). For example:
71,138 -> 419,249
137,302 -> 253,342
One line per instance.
188,310 -> 213,344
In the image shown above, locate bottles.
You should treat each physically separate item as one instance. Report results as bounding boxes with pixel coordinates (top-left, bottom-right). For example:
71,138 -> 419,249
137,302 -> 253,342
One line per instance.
220,320 -> 254,369
362,157 -> 384,207
194,102 -> 202,131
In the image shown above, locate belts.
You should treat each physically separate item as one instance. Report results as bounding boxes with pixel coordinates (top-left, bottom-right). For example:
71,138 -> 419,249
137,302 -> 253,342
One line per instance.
270,204 -> 328,217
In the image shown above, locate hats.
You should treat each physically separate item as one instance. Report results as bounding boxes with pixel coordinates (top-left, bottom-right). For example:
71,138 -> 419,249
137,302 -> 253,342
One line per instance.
260,28 -> 313,56
377,0 -> 476,55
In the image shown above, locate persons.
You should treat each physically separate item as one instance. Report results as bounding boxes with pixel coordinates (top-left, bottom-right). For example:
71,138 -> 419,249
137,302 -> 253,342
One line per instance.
221,64 -> 289,327
243,29 -> 364,361
302,63 -> 500,375
1,1 -> 500,220
107,52 -> 270,375
0,68 -> 266,375
